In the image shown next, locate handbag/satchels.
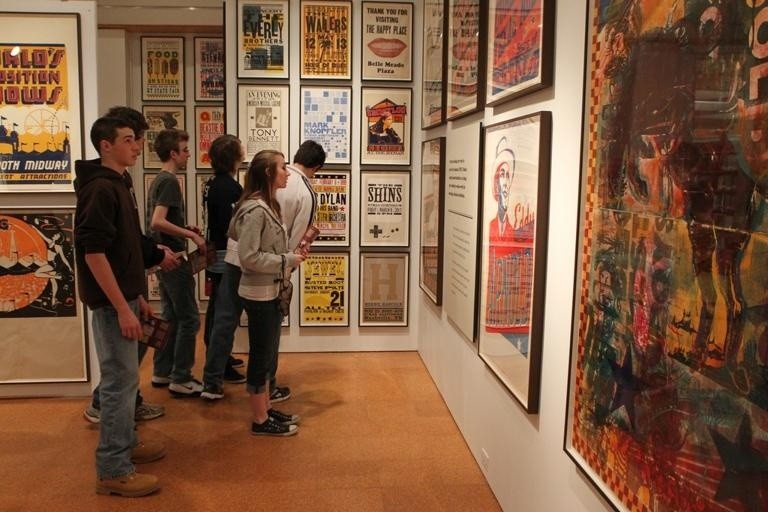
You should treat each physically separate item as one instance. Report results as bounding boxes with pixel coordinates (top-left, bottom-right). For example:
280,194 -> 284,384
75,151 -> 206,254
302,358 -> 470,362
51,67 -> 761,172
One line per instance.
278,278 -> 293,317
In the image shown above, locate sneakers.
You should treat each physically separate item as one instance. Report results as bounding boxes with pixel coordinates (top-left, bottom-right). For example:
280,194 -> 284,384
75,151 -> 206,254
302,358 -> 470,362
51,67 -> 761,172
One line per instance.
83,407 -> 101,424
267,409 -> 301,425
269,386 -> 290,403
221,368 -> 246,384
230,355 -> 244,368
170,379 -> 204,397
151,374 -> 174,388
133,403 -> 165,420
201,383 -> 225,400
252,417 -> 298,436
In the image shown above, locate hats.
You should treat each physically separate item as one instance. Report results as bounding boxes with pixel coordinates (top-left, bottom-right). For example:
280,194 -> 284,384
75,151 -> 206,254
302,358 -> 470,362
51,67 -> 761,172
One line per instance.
489,136 -> 515,199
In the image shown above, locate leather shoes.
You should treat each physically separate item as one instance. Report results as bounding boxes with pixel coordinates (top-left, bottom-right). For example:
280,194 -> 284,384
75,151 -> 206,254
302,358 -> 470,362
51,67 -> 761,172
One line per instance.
130,441 -> 167,463
95,473 -> 160,496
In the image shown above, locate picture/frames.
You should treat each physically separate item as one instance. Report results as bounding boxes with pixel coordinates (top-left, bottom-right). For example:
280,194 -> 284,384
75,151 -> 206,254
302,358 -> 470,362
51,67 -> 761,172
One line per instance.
193,36 -> 225,102
0,203 -> 93,385
444,0 -> 485,121
0,9 -> 87,195
140,34 -> 187,102
360,85 -> 413,166
361,1 -> 415,82
478,110 -> 551,414
299,83 -> 353,164
359,250 -> 411,327
484,0 -> 555,108
298,0 -> 354,82
141,104 -> 187,171
420,0 -> 448,129
235,0 -> 292,82
419,136 -> 447,305
238,310 -> 289,327
306,168 -> 353,247
143,173 -> 188,250
238,168 -> 248,190
237,83 -> 291,164
196,173 -> 216,240
443,122 -> 481,343
198,269 -> 214,301
147,270 -> 160,301
562,0 -> 768,511
297,250 -> 351,328
194,105 -> 225,169
358,169 -> 412,249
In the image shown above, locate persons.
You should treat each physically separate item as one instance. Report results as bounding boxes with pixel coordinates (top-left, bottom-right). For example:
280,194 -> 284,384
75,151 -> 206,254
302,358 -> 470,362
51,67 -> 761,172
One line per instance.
199,138 -> 327,403
31,225 -> 74,307
202,133 -> 249,383
489,137 -> 517,243
368,112 -> 401,144
82,106 -> 182,425
144,129 -> 211,399
230,148 -> 310,437
70,115 -> 169,497
